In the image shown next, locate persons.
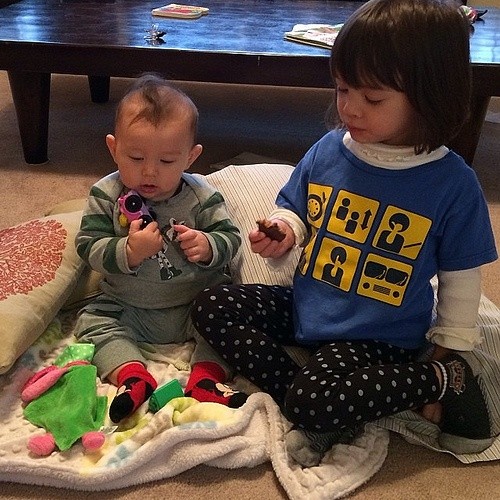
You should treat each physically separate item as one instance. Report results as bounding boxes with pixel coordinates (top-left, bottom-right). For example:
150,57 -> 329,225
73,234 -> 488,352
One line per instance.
188,0 -> 499,466
75,73 -> 248,423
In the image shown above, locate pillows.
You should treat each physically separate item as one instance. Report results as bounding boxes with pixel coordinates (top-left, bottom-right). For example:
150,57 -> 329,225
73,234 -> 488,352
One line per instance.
0,198 -> 104,374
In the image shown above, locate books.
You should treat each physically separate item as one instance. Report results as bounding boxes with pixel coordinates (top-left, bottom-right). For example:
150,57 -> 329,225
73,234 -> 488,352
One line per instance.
152,4 -> 210,19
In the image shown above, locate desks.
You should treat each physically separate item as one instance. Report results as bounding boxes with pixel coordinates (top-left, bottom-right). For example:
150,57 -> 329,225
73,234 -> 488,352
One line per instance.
0,0 -> 500,165
